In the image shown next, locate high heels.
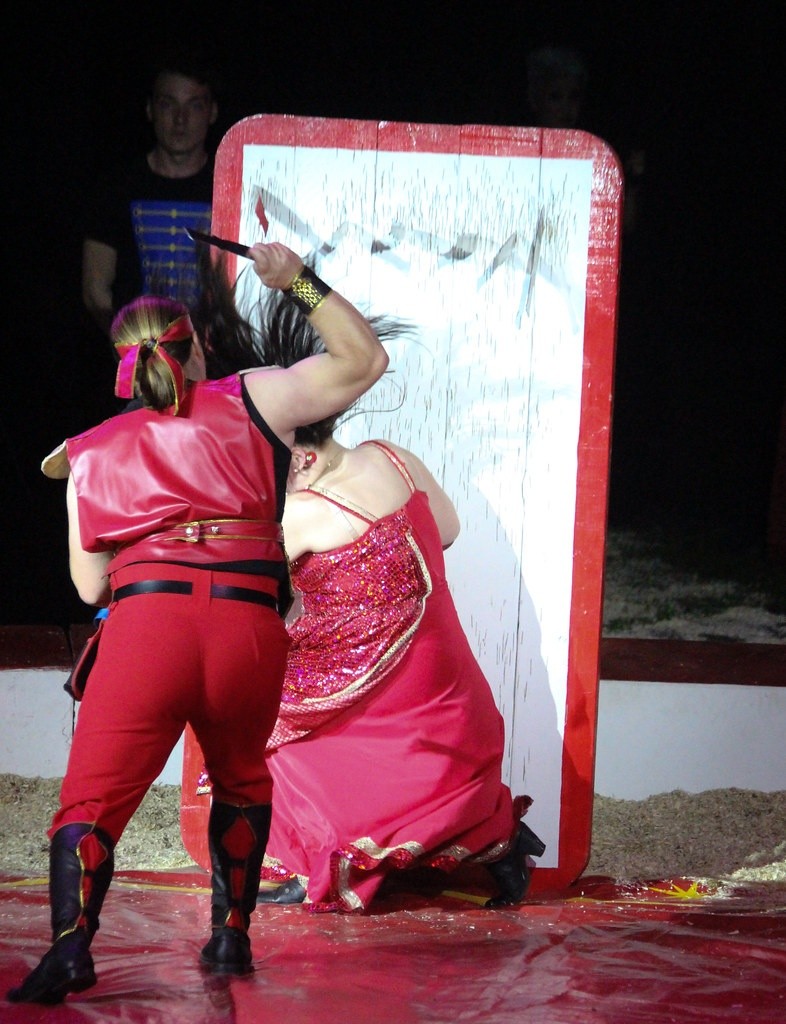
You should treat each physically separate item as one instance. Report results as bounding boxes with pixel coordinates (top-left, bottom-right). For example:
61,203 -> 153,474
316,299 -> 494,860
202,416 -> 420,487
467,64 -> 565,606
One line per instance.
255,875 -> 306,904
482,820 -> 546,908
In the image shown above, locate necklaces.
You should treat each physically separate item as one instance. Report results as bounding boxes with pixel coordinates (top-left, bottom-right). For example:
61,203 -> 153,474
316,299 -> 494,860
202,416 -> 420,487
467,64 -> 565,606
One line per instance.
310,447 -> 345,484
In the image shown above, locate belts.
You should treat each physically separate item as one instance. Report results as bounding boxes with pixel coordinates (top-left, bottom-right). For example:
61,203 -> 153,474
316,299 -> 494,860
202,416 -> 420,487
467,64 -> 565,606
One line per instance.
111,580 -> 277,611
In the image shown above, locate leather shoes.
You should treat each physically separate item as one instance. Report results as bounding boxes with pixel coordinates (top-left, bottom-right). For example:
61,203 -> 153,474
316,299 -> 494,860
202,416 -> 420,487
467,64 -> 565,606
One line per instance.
198,927 -> 252,975
7,937 -> 96,1003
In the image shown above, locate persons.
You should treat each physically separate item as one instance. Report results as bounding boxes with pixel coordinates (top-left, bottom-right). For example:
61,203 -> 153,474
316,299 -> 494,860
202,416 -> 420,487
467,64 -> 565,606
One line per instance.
190,264 -> 548,908
7,242 -> 389,1005
80,66 -> 218,338
